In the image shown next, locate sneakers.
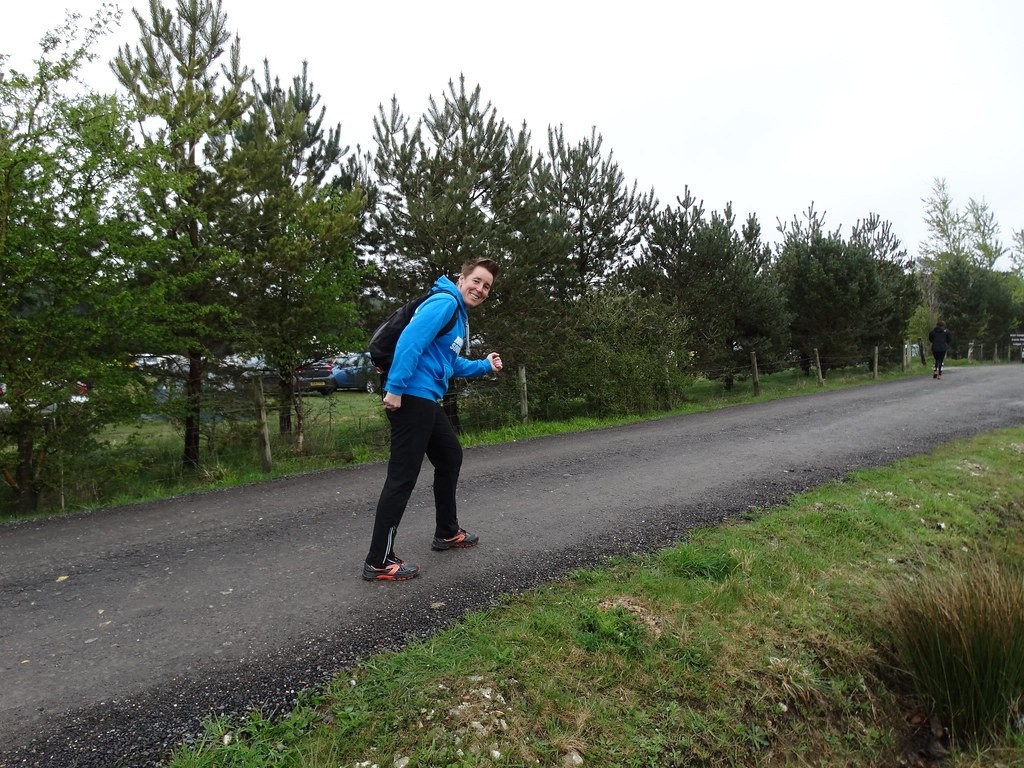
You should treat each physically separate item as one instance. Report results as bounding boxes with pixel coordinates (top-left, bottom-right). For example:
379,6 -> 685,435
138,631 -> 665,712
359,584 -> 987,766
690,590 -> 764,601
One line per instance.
430,526 -> 479,550
361,556 -> 421,580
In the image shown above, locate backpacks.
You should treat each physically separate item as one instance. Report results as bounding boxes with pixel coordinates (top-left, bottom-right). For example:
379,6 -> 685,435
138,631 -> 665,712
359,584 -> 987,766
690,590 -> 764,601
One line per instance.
368,289 -> 459,372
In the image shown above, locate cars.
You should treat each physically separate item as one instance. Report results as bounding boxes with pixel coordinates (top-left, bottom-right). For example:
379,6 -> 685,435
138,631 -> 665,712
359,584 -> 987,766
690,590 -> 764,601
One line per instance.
294,357 -> 335,396
329,352 -> 387,395
0,342 -> 356,418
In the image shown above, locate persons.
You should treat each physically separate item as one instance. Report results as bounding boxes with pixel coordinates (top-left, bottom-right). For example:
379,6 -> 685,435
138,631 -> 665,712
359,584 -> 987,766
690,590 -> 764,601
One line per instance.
929,321 -> 951,379
362,255 -> 503,582
